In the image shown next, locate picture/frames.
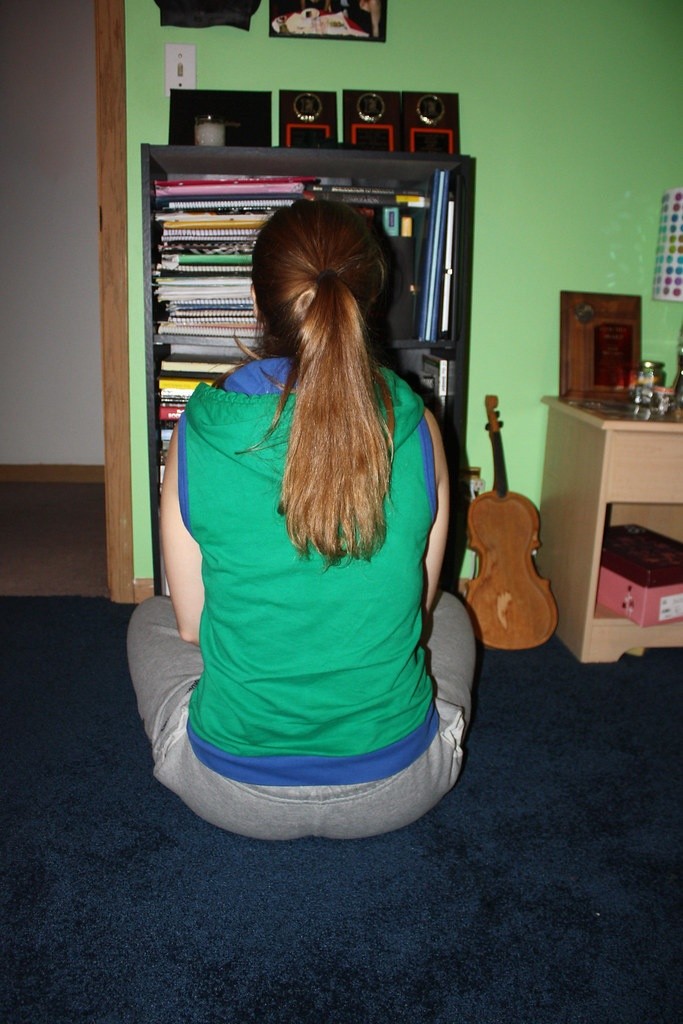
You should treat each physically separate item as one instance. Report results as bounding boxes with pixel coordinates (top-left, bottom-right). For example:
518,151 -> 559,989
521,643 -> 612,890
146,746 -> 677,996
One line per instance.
269,0 -> 387,43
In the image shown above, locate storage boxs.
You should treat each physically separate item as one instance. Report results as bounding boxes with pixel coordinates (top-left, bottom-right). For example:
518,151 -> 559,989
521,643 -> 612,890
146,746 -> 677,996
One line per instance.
596,524 -> 683,628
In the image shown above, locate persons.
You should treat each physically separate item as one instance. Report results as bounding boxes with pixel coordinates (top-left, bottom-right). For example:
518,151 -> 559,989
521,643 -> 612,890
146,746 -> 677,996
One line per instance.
126,199 -> 475,842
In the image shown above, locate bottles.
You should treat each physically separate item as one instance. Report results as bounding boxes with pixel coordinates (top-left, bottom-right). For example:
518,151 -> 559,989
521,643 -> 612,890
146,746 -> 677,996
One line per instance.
632,360 -> 676,418
194,114 -> 225,146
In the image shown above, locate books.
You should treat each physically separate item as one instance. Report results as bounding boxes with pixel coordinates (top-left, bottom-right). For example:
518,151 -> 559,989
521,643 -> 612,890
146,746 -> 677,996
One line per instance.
150,167 -> 455,514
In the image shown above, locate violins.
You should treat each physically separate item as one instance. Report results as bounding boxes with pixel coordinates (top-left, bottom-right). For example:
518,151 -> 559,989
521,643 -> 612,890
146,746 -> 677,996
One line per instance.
465,396 -> 558,651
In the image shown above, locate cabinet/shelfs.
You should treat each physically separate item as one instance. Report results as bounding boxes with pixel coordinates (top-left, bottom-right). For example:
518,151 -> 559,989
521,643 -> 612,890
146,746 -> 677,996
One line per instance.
534,396 -> 683,663
141,144 -> 477,597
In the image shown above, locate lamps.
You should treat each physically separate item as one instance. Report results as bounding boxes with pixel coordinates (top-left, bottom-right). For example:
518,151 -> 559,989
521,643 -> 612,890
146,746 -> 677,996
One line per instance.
653,188 -> 683,407
